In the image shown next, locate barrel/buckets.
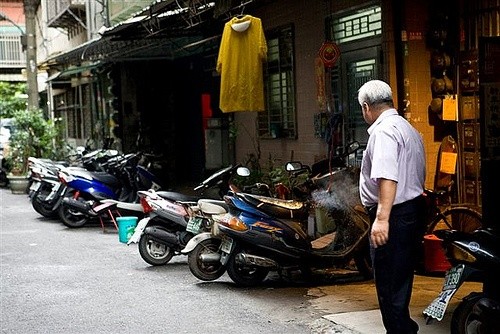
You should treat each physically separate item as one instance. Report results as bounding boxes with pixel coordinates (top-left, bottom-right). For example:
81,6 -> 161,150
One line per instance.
116,216 -> 138,243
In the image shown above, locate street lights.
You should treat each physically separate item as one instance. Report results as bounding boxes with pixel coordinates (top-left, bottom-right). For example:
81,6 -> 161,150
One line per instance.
0,14 -> 41,160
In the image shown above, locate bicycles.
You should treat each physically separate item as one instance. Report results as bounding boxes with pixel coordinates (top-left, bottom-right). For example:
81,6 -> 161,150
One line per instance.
424,189 -> 484,282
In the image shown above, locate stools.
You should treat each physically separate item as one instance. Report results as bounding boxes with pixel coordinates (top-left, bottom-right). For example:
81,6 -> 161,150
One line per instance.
93,202 -> 124,233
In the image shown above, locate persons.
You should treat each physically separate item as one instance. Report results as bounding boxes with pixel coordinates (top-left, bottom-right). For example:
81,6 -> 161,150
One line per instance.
357,79 -> 426,334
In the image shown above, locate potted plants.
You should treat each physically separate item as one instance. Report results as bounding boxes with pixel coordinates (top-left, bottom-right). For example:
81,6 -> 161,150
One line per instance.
0,81 -> 65,194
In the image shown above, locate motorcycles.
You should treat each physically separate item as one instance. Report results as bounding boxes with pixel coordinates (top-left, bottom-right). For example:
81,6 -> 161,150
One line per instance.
421,223 -> 500,334
28,136 -> 373,287
